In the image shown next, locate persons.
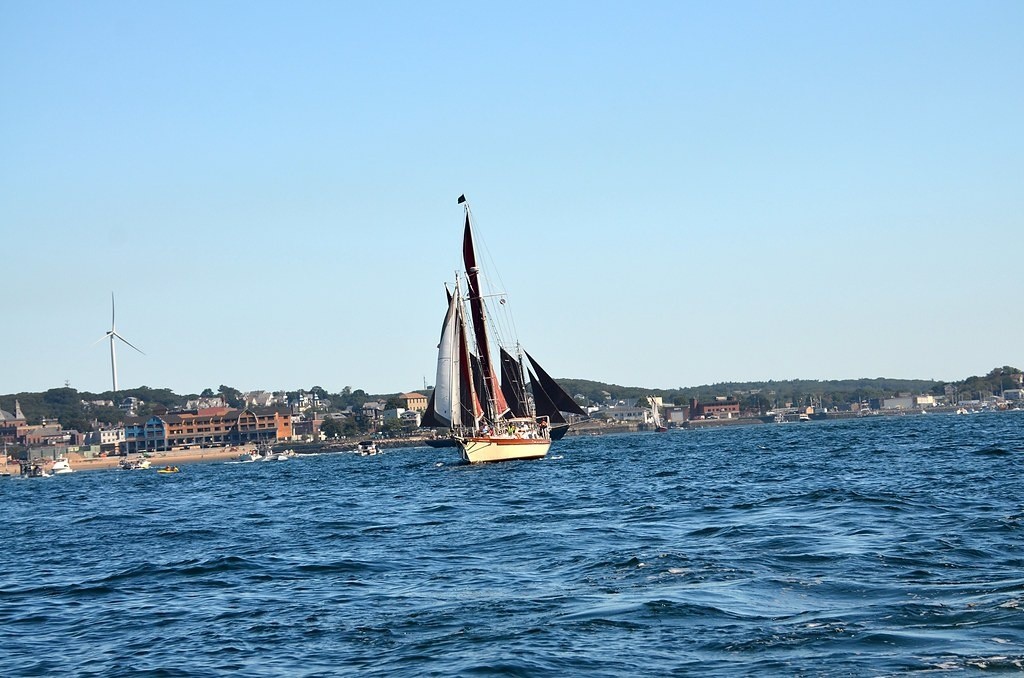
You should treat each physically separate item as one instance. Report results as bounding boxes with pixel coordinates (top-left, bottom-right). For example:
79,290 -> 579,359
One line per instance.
508,423 -> 515,435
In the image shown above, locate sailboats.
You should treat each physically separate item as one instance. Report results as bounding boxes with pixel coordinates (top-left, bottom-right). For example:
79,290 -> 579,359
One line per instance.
420,192 -> 590,462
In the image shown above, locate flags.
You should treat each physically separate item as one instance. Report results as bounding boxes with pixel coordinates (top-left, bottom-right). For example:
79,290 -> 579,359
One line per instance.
458,194 -> 466,204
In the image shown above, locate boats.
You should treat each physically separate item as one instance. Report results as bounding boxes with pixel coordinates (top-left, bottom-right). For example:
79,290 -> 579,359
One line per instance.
655,426 -> 668,432
2,456 -> 74,478
355,440 -> 378,457
118,457 -> 152,471
767,402 -> 1022,416
157,467 -> 180,473
592,432 -> 602,435
246,448 -> 296,462
